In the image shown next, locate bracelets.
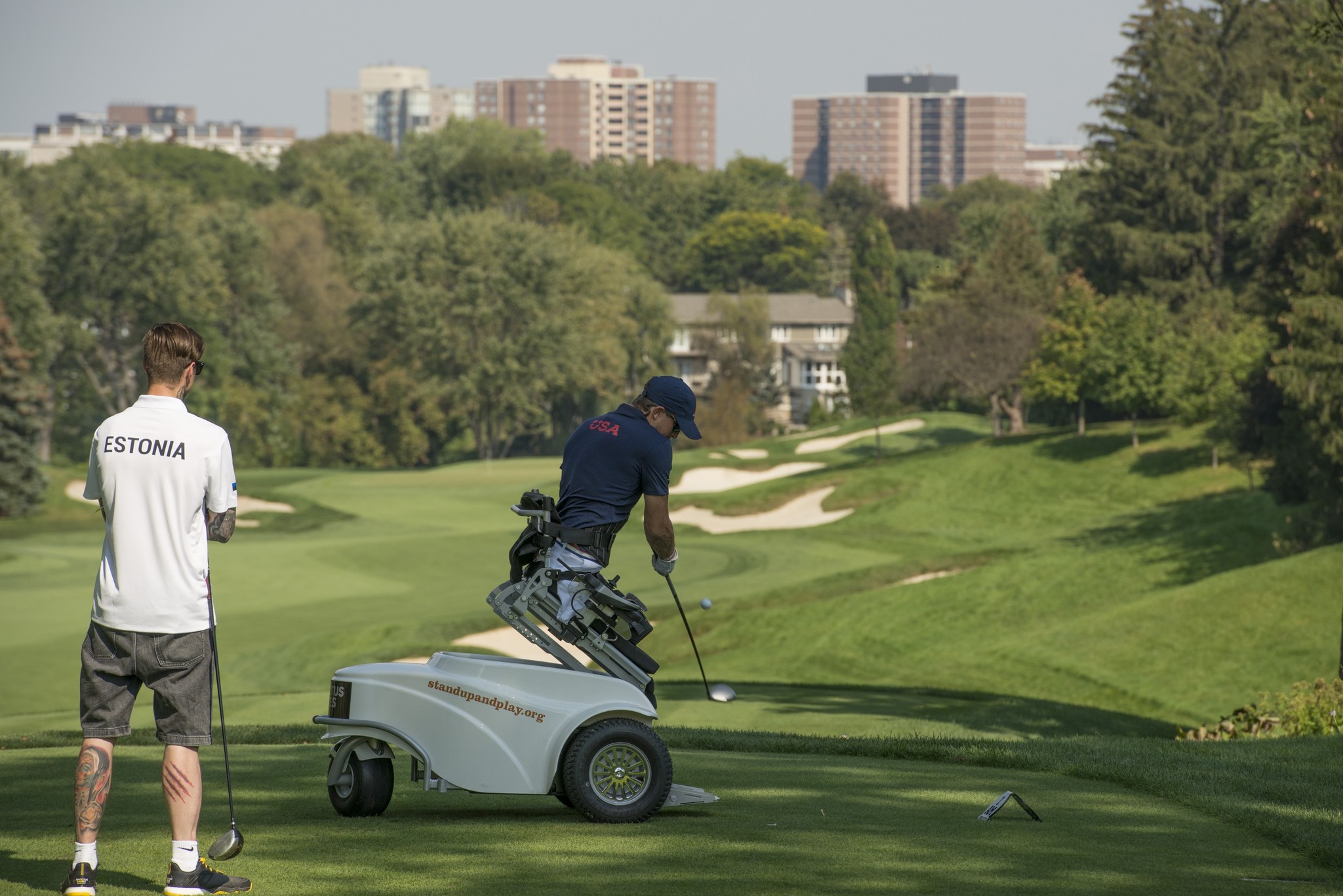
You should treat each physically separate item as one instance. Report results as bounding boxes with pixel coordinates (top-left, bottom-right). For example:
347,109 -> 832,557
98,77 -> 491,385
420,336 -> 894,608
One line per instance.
663,548 -> 675,560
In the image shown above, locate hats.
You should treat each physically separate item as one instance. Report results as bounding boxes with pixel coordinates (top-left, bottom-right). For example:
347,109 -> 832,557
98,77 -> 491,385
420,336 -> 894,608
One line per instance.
642,376 -> 702,440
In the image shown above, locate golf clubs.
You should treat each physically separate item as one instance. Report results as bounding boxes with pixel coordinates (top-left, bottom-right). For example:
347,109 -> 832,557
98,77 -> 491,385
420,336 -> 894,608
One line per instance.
205,565 -> 245,861
663,573 -> 737,703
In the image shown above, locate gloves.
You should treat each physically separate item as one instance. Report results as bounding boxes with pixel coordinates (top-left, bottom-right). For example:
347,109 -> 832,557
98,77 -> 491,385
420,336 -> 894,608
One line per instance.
652,548 -> 678,576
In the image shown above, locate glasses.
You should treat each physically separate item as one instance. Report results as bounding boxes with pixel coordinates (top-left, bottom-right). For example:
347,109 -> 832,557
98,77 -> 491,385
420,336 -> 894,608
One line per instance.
184,361 -> 204,375
647,404 -> 681,431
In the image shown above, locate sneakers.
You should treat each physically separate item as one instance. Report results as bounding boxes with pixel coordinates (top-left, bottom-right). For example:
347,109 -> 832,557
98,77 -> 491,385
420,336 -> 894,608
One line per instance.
60,862 -> 98,896
164,856 -> 252,896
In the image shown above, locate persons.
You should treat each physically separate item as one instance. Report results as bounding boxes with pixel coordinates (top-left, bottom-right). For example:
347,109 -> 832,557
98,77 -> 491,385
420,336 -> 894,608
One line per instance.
547,375 -> 702,625
60,322 -> 252,896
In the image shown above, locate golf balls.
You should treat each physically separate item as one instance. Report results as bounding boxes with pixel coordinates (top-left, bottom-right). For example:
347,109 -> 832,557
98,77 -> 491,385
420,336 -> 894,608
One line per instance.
700,598 -> 712,610
